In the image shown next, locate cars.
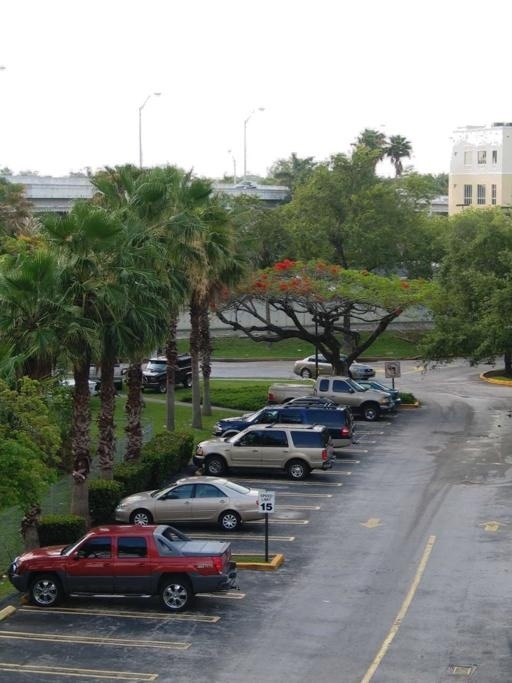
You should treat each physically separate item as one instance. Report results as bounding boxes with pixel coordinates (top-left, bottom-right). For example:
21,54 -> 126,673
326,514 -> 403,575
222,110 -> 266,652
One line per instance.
233,179 -> 256,190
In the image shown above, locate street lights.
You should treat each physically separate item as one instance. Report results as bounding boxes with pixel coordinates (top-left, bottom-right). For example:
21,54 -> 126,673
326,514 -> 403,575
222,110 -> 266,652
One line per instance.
244,107 -> 265,179
227,149 -> 237,184
139,92 -> 161,170
